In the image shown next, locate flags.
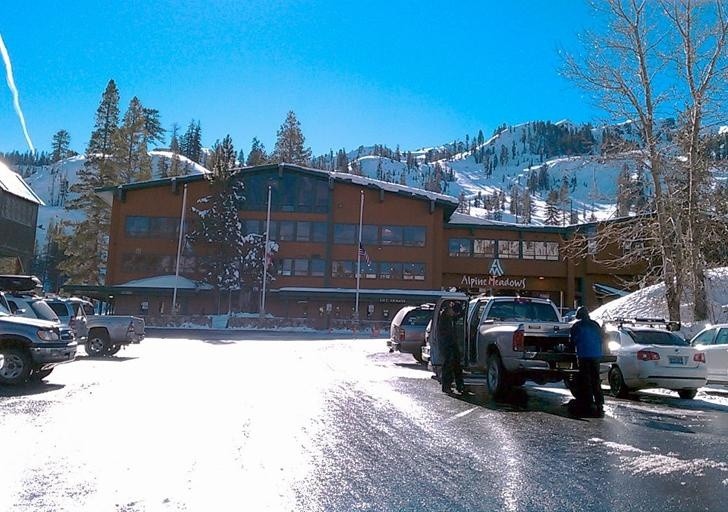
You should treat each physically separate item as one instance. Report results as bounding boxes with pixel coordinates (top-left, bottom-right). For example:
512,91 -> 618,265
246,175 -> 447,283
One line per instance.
358,243 -> 370,262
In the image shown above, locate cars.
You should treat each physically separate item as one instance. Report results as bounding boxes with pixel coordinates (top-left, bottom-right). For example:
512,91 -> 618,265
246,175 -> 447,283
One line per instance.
690,324 -> 727,385
385,304 -> 431,364
43,297 -> 89,339
598,319 -> 708,399
0,275 -> 77,385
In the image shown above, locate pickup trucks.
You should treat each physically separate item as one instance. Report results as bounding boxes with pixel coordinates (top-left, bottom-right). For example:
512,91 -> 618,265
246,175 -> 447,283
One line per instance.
420,297 -> 577,401
81,301 -> 145,357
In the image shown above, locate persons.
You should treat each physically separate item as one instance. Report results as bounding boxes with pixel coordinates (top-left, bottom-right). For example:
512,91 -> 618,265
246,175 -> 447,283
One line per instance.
436,303 -> 468,396
84,302 -> 93,315
569,306 -> 605,419
